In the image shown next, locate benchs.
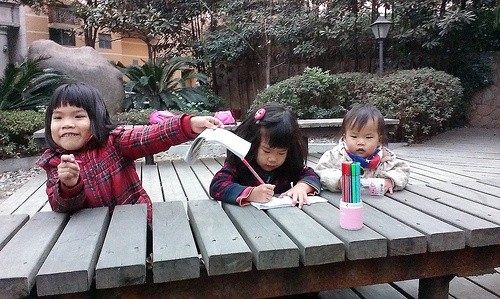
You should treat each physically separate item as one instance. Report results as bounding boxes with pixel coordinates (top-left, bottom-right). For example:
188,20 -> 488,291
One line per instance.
33,118 -> 400,165
0,180 -> 500,299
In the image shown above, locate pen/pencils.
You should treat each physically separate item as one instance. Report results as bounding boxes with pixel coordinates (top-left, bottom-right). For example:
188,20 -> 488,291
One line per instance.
241,158 -> 265,185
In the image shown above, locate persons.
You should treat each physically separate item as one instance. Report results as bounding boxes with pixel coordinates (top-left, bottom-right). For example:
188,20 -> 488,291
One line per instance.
208,104 -> 322,213
33,80 -> 225,246
313,101 -> 413,197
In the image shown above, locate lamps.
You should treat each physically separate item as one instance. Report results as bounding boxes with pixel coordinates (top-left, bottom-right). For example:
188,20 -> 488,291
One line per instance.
370,16 -> 393,43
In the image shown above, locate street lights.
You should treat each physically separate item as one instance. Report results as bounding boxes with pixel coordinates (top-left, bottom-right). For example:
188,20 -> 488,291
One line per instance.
369,6 -> 391,78
